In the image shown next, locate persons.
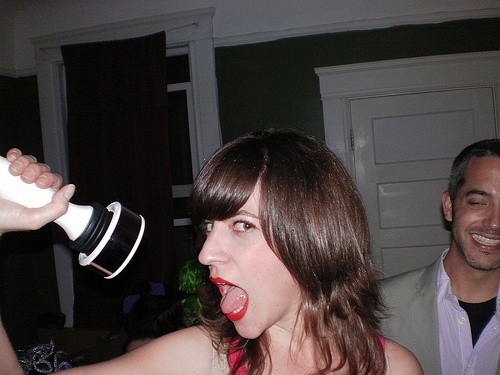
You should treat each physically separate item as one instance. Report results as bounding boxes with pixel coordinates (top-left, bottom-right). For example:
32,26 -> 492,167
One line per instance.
1,130 -> 425,374
373,139 -> 500,375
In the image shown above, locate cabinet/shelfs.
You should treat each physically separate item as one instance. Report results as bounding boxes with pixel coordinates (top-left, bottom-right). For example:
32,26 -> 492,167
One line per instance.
315,50 -> 500,281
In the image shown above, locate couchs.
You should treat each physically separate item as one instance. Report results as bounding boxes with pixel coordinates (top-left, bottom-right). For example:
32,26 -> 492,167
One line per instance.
35,326 -> 128,369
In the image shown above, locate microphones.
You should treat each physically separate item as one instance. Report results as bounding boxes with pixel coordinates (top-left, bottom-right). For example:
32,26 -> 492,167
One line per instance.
0,156 -> 153,281
